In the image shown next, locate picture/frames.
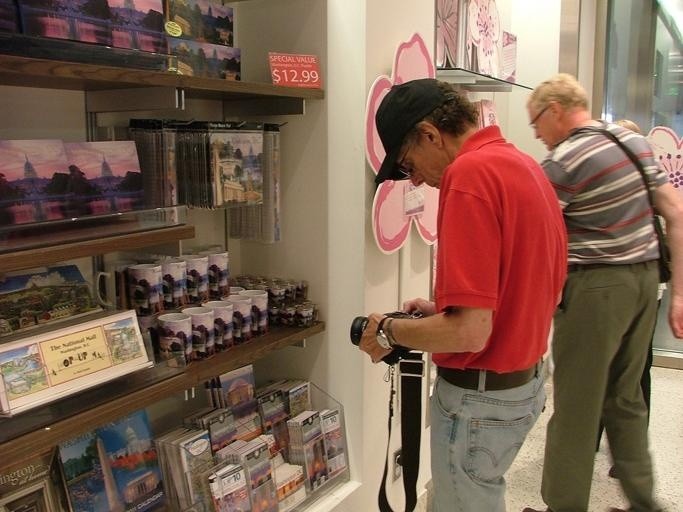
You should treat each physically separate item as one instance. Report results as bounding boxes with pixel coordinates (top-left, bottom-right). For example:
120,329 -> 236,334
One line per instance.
1,445 -> 75,512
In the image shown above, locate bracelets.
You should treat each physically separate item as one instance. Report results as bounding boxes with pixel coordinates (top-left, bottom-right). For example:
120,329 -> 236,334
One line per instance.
386,318 -> 398,345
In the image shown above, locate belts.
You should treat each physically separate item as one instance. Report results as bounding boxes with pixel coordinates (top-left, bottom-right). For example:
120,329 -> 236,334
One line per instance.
566,261 -> 610,270
437,364 -> 537,392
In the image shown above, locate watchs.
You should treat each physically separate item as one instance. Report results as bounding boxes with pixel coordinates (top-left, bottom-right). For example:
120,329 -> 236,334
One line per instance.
375,317 -> 412,351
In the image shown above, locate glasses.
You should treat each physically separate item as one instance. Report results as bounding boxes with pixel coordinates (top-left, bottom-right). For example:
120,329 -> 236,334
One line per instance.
397,134 -> 416,176
527,106 -> 548,128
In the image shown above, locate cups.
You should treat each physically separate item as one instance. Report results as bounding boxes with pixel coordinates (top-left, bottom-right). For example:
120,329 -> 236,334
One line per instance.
96,250 -> 267,369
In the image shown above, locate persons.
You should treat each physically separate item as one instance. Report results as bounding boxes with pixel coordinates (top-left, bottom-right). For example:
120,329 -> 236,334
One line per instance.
595,119 -> 670,478
357,78 -> 568,511
525,72 -> 682,512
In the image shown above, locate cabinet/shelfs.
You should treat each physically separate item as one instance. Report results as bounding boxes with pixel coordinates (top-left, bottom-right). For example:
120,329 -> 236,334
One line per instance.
1,50 -> 362,512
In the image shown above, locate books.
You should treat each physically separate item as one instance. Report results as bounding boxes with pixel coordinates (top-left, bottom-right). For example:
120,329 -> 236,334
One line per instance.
149,362 -> 351,512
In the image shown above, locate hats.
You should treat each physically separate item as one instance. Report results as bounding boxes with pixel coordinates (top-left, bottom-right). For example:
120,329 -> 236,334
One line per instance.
375,79 -> 458,185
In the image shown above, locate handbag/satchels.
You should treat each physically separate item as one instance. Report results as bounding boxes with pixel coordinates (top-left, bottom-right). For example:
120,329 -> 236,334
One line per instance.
652,215 -> 669,283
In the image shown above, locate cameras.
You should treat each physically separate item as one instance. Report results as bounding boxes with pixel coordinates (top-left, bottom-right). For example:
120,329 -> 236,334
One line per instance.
351,312 -> 424,367
